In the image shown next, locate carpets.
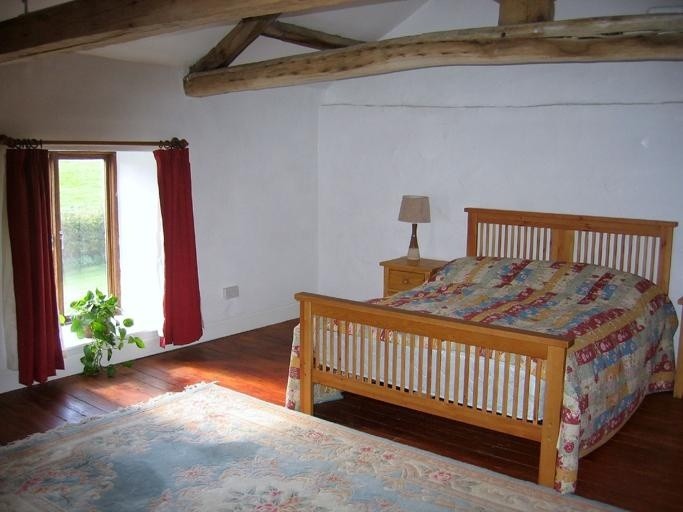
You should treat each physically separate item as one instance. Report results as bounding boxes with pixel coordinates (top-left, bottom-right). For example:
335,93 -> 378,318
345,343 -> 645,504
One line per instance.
1,381 -> 634,511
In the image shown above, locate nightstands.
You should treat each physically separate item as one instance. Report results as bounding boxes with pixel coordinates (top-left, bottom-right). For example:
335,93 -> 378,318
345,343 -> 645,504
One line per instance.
378,257 -> 448,297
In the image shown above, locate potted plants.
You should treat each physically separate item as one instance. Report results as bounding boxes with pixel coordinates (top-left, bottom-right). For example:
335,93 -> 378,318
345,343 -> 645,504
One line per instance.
58,287 -> 146,377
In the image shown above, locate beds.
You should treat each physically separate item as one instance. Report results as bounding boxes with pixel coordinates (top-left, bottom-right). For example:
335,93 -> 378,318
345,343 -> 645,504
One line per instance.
294,207 -> 680,495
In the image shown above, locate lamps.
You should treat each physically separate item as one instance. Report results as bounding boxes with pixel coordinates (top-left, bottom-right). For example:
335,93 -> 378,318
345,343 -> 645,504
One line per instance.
396,195 -> 430,260
